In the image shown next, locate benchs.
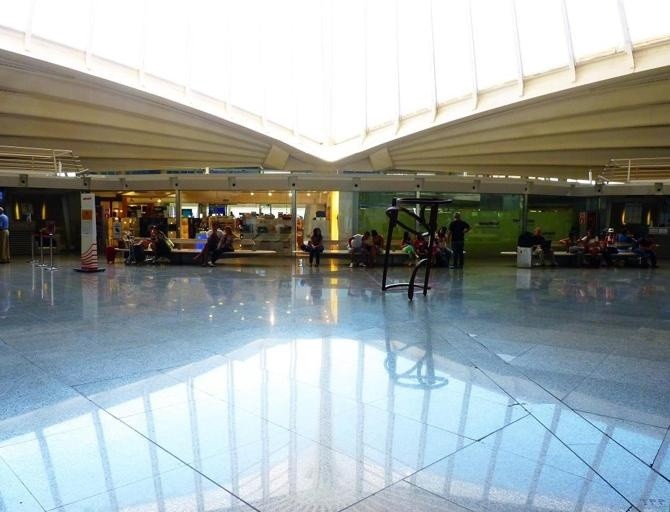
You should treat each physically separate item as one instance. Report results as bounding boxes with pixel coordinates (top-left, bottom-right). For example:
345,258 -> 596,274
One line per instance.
292,240 -> 467,266
500,240 -> 660,267
114,238 -> 278,264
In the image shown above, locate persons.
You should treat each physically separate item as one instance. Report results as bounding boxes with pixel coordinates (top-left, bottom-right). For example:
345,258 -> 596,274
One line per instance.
0,207 -> 11,263
400,226 -> 453,266
309,228 -> 324,267
448,212 -> 472,269
532,227 -> 546,266
560,228 -> 658,268
202,223 -> 236,267
349,229 -> 384,268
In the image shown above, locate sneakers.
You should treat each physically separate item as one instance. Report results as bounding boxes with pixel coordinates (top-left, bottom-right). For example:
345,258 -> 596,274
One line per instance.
349,262 -> 367,268
